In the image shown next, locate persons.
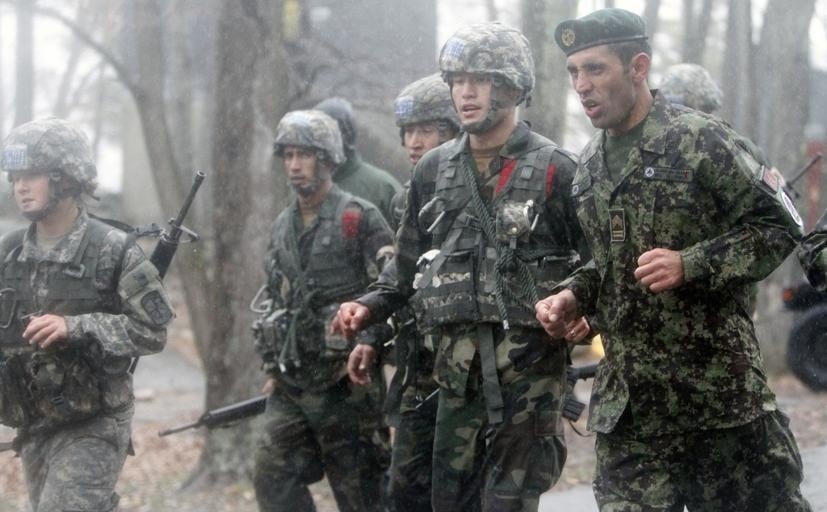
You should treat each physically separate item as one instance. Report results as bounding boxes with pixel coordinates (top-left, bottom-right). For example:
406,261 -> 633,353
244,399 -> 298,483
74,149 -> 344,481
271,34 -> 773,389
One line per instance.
795,207 -> 826,298
314,96 -> 403,232
325,18 -> 603,511
533,7 -> 816,512
248,109 -> 397,512
0,116 -> 174,511
653,64 -> 726,122
345,72 -> 463,512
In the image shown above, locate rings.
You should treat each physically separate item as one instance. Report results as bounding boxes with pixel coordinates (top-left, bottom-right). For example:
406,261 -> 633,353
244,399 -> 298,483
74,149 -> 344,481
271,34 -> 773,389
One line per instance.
569,328 -> 578,340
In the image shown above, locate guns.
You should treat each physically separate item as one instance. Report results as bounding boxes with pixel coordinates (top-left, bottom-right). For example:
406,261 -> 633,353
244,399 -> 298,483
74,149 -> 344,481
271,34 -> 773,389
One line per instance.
561,364 -> 599,421
160,337 -> 398,435
125,172 -> 206,455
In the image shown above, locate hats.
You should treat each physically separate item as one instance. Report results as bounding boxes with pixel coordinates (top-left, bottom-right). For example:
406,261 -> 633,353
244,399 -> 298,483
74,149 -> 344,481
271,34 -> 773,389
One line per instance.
553,8 -> 648,56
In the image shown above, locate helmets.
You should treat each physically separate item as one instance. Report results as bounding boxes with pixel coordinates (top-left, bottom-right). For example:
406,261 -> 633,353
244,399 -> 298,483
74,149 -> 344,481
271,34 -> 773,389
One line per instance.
394,72 -> 460,130
659,63 -> 723,112
274,109 -> 346,166
313,97 -> 356,141
438,22 -> 535,90
1,119 -> 97,183
